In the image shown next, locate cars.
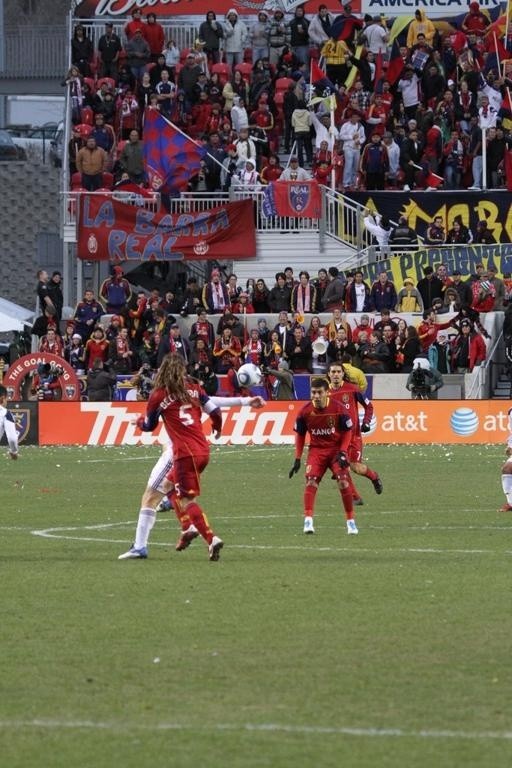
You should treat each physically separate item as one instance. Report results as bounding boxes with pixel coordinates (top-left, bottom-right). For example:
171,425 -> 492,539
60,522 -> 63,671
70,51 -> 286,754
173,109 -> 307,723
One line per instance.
0,120 -> 65,168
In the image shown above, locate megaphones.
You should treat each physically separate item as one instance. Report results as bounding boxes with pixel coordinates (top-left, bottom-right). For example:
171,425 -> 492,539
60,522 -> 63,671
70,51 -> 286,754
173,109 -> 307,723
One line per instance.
312,335 -> 328,355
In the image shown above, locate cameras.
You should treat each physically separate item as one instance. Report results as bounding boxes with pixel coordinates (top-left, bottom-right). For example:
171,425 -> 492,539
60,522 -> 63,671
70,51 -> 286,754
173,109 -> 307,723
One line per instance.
142,368 -> 152,378
103,358 -> 113,368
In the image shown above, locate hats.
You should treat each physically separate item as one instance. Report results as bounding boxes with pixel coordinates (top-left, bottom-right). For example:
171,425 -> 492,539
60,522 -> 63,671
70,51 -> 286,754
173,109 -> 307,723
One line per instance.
460,317 -> 472,328
479,280 -> 493,294
277,360 -> 289,370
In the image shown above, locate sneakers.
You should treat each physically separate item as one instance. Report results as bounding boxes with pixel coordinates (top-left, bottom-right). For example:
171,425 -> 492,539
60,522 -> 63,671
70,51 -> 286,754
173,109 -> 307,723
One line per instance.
175,523 -> 200,552
303,516 -> 314,534
371,470 -> 383,495
354,496 -> 365,506
346,518 -> 359,536
497,503 -> 511,513
155,495 -> 174,513
207,536 -> 224,562
116,542 -> 149,562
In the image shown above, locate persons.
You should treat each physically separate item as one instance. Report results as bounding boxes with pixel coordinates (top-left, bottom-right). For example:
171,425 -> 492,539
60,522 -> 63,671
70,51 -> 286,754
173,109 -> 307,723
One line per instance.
130,352 -> 225,563
288,377 -> 361,536
495,407 -> 512,514
1,0 -> 511,402
1,383 -> 21,461
325,361 -> 383,506
116,421 -> 175,561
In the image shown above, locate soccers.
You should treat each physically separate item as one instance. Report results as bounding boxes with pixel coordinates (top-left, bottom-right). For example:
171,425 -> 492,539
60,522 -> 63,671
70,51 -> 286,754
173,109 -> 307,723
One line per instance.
354,411 -> 375,435
236,363 -> 262,389
450,410 -> 478,435
